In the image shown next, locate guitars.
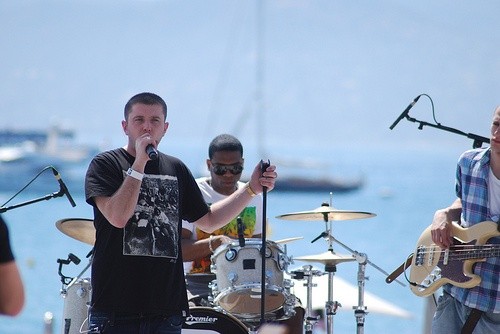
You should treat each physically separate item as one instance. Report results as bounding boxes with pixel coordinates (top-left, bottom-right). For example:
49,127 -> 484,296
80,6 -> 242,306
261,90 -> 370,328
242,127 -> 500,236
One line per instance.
409,219 -> 500,298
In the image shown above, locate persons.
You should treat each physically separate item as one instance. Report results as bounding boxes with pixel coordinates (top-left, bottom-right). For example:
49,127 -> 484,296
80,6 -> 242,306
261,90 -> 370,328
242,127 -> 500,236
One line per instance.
84,93 -> 277,334
0,216 -> 25,316
180,134 -> 269,276
431,105 -> 500,334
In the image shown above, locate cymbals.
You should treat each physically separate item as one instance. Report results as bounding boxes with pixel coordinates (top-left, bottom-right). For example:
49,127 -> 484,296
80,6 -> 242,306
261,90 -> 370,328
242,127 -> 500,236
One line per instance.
275,236 -> 304,245
290,251 -> 357,264
55,216 -> 97,247
274,207 -> 376,221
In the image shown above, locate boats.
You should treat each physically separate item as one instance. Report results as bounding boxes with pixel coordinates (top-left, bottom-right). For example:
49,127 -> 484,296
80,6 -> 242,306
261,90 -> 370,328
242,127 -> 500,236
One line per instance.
194,1 -> 364,194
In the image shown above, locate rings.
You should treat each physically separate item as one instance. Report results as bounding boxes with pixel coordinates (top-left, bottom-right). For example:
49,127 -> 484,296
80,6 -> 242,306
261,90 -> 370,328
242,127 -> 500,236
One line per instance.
147,137 -> 150,140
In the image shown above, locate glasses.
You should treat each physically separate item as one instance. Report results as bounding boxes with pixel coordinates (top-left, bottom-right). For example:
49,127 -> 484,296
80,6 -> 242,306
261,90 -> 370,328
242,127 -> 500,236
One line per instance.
210,160 -> 243,175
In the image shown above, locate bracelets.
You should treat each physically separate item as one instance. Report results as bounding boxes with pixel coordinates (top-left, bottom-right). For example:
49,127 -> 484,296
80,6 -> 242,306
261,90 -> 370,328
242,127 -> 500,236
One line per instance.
246,181 -> 256,197
209,235 -> 215,253
126,168 -> 143,181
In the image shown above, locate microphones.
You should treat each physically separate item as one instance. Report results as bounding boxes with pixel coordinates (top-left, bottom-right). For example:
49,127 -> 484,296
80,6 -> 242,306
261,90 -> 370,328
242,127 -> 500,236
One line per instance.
145,144 -> 158,159
390,95 -> 420,129
52,168 -> 76,207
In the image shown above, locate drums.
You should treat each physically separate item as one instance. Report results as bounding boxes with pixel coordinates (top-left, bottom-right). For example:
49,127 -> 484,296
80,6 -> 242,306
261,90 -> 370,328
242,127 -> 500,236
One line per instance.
180,305 -> 251,334
60,277 -> 93,334
210,237 -> 287,316
